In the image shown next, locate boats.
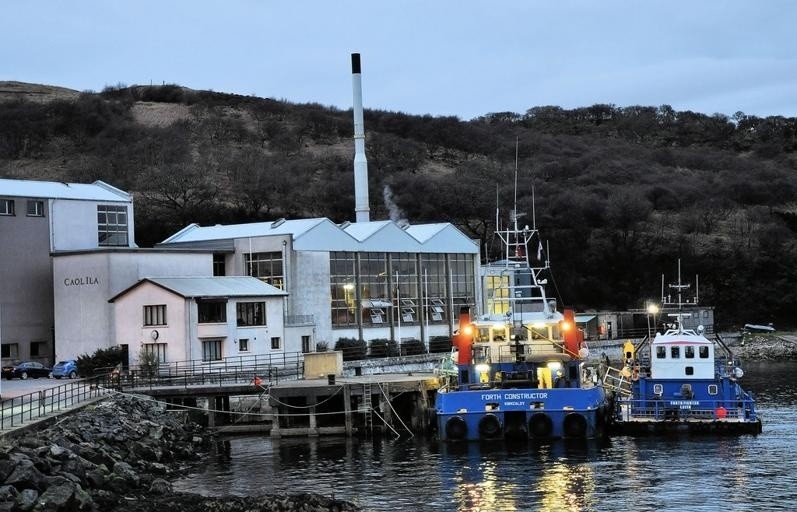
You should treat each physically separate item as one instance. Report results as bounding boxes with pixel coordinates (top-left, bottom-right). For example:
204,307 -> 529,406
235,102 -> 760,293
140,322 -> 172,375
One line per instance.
620,259 -> 762,435
434,135 -> 615,443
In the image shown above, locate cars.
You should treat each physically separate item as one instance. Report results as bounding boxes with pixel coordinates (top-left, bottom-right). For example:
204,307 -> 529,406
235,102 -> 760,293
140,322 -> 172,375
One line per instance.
2,360 -> 77,379
